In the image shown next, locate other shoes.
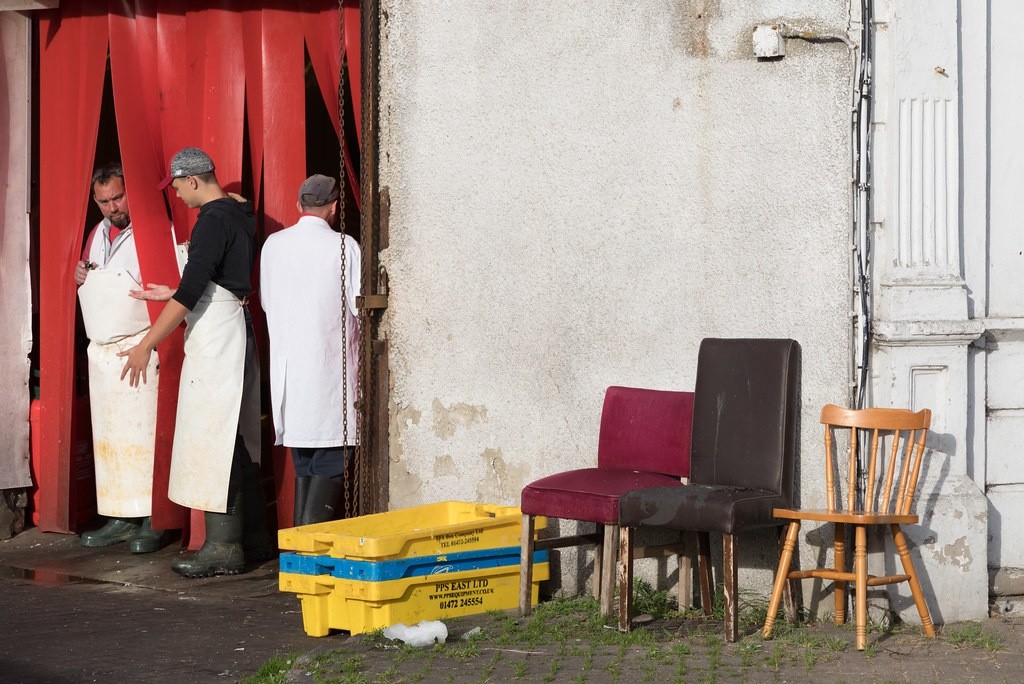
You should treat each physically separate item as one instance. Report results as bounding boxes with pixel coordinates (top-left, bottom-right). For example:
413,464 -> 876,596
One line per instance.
79,516 -> 141,547
130,517 -> 178,553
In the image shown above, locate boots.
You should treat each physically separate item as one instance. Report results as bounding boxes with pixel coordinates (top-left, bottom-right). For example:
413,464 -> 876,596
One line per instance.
172,491 -> 246,577
295,475 -> 311,526
302,476 -> 343,525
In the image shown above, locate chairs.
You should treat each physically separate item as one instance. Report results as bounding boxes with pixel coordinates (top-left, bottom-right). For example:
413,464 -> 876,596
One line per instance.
764,402 -> 934,653
522,385 -> 697,620
617,337 -> 805,643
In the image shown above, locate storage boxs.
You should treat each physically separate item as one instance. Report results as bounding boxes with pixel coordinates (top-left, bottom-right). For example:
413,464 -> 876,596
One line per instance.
275,501 -> 551,639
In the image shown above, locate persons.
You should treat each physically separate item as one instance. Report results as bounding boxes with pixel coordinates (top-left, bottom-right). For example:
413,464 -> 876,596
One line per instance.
74,161 -> 176,552
116,148 -> 261,579
260,175 -> 361,525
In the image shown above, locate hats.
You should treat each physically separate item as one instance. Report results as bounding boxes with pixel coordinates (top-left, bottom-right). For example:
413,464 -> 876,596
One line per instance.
298,174 -> 339,206
157,147 -> 216,191
